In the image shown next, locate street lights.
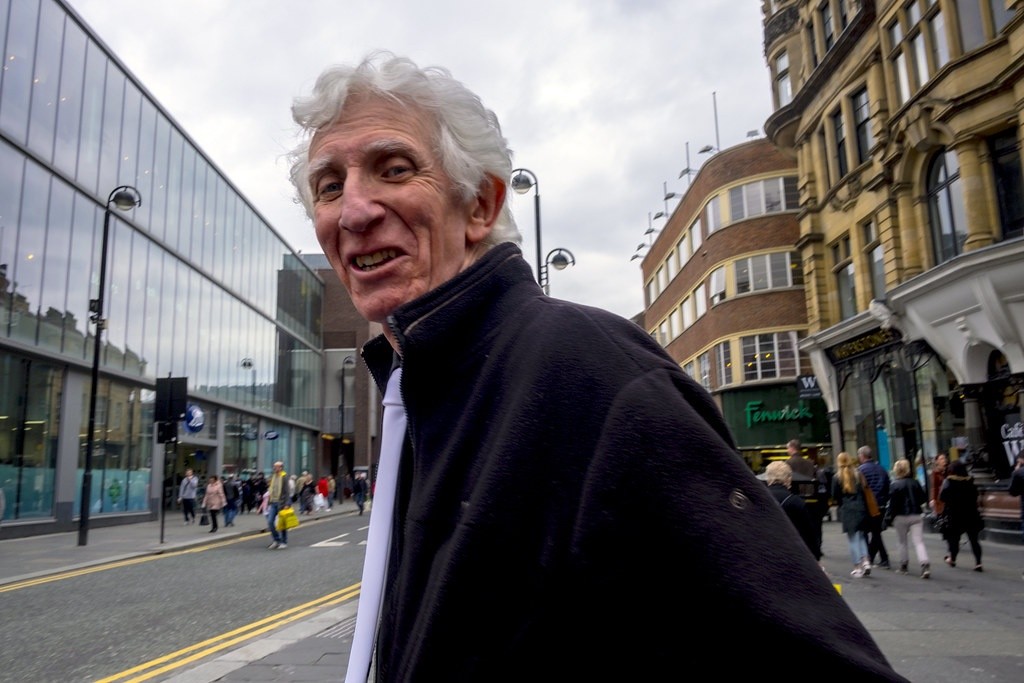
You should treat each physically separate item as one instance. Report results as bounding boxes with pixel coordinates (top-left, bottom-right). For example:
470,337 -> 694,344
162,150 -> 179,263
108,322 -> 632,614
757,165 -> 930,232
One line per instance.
509,168 -> 543,290
77,184 -> 144,546
338,354 -> 359,504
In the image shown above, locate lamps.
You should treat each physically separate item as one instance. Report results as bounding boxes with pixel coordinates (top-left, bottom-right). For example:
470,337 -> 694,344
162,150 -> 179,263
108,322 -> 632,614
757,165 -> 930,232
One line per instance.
644,229 -> 659,235
636,243 -> 651,252
679,168 -> 698,179
664,192 -> 683,201
747,130 -> 759,137
630,255 -> 644,261
653,213 -> 671,220
698,145 -> 720,155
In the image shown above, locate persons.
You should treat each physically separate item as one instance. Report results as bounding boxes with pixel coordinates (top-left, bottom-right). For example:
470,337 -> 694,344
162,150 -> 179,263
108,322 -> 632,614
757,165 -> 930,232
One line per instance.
285,50 -> 911,683
178,468 -> 199,524
266,461 -> 291,549
762,439 -> 986,577
288,470 -> 375,516
221,471 -> 272,527
1008,449 -> 1024,521
201,475 -> 227,532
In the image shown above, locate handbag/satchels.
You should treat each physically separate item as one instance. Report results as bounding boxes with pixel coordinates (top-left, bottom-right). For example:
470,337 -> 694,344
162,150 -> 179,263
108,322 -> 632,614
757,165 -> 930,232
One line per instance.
859,472 -> 881,517
200,507 -> 209,525
275,507 -> 300,531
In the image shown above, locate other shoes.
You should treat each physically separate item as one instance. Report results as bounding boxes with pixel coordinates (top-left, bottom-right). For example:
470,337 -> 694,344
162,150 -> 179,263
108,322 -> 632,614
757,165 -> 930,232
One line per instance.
973,564 -> 982,572
862,561 -> 871,576
944,556 -> 956,567
268,541 -> 279,549
276,543 -> 287,549
210,528 -> 217,532
920,565 -> 931,579
895,565 -> 909,575
872,560 -> 890,569
850,569 -> 863,578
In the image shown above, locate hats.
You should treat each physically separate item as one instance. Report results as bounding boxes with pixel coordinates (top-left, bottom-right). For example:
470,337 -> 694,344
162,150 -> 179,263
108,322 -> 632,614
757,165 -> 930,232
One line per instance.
755,461 -> 792,480
